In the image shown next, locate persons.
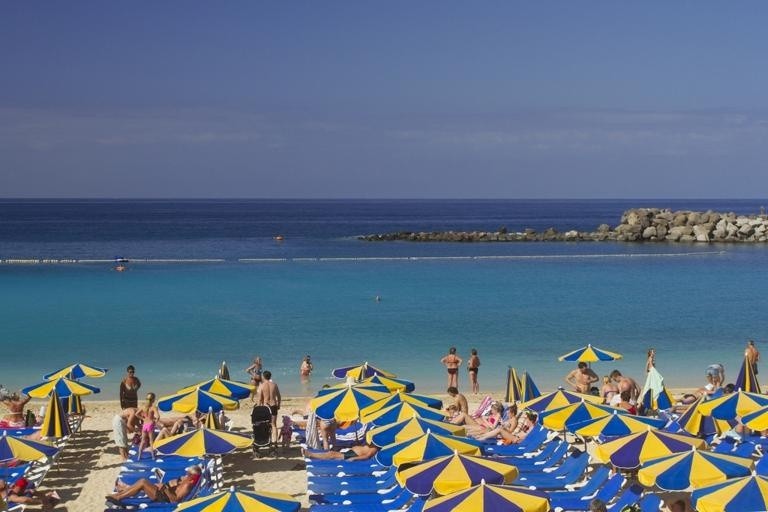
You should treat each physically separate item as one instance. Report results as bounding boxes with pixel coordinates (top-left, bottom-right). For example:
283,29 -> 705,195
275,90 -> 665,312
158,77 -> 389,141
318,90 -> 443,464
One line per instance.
1,392 -> 61,505
441,340 -> 766,446
245,355 -> 383,462
104,361 -> 231,504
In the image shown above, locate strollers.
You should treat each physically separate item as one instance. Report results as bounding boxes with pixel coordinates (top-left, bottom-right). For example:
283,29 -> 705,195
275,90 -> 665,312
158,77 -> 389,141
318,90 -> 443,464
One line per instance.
248,403 -> 282,459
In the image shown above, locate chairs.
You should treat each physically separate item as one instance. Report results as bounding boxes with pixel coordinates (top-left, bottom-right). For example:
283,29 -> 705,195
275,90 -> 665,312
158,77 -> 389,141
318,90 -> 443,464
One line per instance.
106,408 -> 230,512
1,411 -> 88,511
292,412 -> 430,511
661,421 -> 768,481
479,407 -> 661,511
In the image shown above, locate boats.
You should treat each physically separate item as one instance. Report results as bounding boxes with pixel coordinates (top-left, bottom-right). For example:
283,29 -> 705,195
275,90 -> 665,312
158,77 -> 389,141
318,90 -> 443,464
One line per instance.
110,265 -> 128,272
273,235 -> 283,240
114,255 -> 128,262
